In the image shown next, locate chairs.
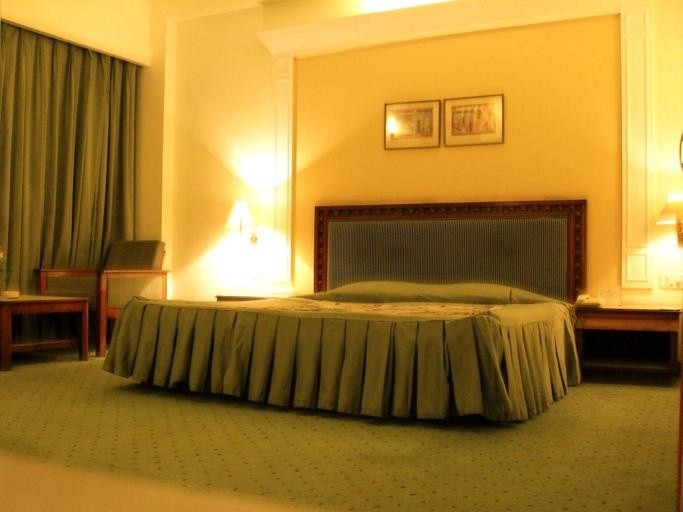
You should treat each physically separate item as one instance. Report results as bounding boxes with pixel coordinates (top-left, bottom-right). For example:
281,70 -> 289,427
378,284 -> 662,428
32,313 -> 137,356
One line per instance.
34,239 -> 169,358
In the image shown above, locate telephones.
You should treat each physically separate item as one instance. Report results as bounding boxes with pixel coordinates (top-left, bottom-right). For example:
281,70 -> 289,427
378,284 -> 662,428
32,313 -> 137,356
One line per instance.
576,294 -> 603,308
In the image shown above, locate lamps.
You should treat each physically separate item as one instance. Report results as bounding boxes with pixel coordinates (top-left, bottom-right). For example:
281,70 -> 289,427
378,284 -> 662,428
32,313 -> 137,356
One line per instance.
655,193 -> 683,256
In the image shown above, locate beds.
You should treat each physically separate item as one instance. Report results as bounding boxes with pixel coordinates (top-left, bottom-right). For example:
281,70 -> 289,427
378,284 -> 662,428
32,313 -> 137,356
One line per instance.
101,197 -> 588,426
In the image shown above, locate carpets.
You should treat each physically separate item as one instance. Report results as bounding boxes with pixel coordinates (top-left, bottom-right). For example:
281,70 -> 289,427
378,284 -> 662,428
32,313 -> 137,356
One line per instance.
0,353 -> 682,512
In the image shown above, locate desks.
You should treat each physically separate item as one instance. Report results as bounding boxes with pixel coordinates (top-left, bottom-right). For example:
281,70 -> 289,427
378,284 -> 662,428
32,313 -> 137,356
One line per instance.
0,291 -> 90,371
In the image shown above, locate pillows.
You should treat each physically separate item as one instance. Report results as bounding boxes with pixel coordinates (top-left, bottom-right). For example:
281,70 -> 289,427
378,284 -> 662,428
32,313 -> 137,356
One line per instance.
326,279 -> 537,303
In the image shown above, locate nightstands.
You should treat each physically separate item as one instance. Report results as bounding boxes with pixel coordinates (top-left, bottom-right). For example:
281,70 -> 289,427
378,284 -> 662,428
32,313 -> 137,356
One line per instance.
575,302 -> 683,387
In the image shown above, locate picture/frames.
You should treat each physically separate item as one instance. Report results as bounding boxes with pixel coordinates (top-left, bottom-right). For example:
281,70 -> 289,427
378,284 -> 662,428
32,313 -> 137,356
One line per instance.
383,93 -> 504,151
